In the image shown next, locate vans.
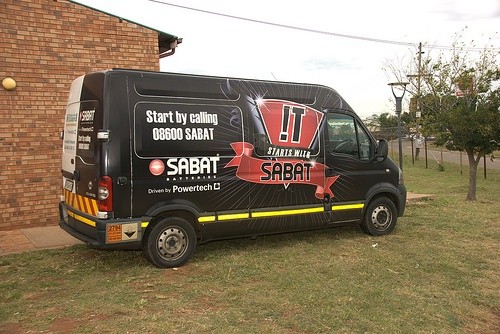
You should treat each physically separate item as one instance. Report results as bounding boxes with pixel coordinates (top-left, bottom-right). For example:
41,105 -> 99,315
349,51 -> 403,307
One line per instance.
59,68 -> 407,268
